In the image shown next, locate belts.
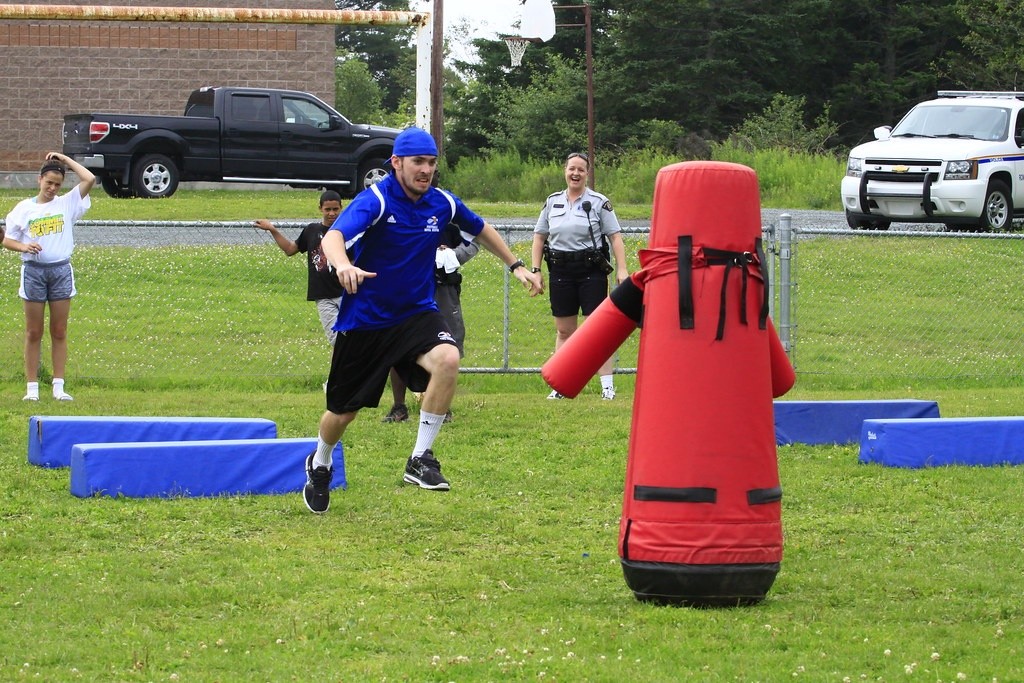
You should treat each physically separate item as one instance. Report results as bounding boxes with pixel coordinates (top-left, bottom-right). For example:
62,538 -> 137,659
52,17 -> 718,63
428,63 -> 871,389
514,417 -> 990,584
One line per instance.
549,250 -> 587,263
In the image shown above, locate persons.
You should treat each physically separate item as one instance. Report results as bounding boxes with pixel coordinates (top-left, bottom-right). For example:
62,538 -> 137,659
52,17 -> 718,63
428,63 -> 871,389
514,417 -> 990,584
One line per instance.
528,152 -> 631,402
298,128 -> 547,512
253,188 -> 357,396
2,151 -> 96,404
379,169 -> 479,424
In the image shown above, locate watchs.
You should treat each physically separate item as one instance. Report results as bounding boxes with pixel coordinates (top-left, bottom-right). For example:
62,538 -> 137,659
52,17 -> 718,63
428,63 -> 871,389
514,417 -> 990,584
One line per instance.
529,266 -> 542,274
506,258 -> 526,273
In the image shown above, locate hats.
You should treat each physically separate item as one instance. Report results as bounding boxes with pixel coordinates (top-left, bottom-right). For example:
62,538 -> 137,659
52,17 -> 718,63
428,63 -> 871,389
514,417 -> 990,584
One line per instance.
384,127 -> 440,166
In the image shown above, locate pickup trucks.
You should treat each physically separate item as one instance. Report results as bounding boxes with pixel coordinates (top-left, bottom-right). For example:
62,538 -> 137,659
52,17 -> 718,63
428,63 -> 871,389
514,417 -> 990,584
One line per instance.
63,84 -> 404,200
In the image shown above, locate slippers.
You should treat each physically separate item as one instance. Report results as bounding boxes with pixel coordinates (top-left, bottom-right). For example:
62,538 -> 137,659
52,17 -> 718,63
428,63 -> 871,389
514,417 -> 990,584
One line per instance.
23,395 -> 40,402
54,393 -> 74,401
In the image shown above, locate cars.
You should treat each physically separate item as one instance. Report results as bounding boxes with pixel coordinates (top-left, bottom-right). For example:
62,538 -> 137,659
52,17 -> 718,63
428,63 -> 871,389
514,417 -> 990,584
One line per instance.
840,91 -> 1024,232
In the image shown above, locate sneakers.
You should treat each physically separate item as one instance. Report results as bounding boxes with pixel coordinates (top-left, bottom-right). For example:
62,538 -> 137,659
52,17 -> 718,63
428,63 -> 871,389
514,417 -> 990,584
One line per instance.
382,406 -> 410,422
547,390 -> 565,400
302,450 -> 333,513
442,411 -> 454,424
602,388 -> 616,400
404,450 -> 451,491
